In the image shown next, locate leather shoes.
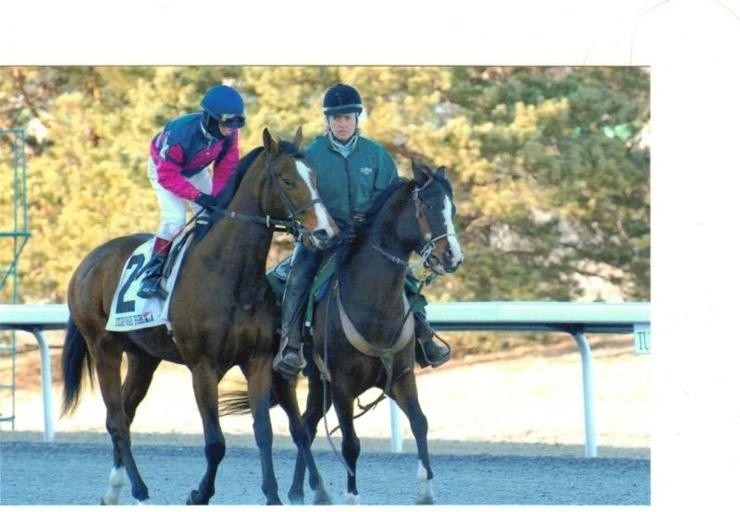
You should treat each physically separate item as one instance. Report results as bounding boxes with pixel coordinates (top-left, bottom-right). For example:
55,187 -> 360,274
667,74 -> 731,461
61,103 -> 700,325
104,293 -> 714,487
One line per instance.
272,347 -> 301,377
415,337 -> 450,368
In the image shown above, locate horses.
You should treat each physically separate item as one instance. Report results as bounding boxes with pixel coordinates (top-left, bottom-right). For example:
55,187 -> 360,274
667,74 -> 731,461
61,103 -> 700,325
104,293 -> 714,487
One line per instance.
56,127 -> 341,505
218,157 -> 464,506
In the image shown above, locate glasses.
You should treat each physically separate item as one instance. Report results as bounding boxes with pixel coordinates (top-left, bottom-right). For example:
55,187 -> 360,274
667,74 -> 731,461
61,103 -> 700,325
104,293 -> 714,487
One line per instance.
219,116 -> 245,129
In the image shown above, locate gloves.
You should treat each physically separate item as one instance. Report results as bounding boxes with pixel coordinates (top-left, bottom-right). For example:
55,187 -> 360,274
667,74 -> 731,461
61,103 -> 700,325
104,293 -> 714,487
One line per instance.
194,191 -> 218,210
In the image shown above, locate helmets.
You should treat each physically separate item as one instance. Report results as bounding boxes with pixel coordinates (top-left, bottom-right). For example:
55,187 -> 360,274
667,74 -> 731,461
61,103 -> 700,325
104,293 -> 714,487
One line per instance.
322,83 -> 362,116
199,84 -> 246,130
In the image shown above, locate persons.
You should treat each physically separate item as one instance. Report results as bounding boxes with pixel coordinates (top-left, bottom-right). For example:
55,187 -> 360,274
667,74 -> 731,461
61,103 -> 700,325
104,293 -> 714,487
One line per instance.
270,83 -> 450,380
135,84 -> 248,298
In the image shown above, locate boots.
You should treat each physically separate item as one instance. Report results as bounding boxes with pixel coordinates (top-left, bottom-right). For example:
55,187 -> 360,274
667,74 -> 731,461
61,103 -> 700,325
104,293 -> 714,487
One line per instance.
137,250 -> 166,298
